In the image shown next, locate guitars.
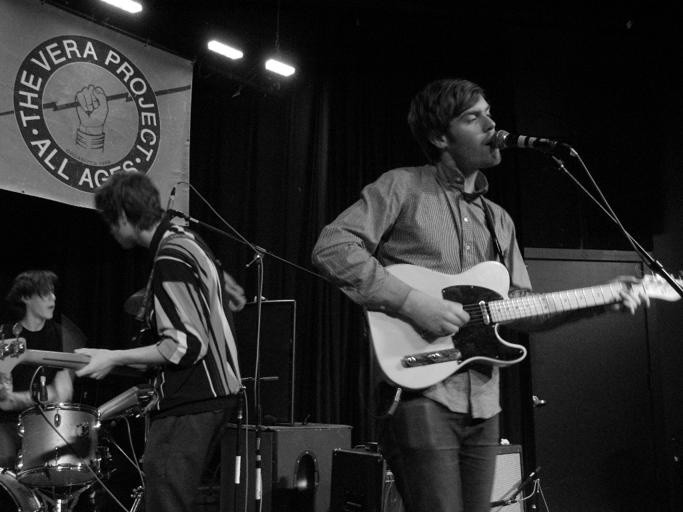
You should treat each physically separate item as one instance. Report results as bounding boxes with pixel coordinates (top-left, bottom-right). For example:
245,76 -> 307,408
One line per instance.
363,261 -> 683,391
0,338 -> 159,382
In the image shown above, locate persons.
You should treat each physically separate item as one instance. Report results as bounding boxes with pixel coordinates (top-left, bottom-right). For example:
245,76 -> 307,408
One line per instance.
0,270 -> 73,412
74,170 -> 242,512
311,79 -> 651,512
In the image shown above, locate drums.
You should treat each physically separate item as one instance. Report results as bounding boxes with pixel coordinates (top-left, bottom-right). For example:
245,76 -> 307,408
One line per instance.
16,402 -> 102,487
1,466 -> 43,511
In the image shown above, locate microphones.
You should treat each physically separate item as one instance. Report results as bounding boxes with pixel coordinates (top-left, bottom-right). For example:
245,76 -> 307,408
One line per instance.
491,128 -> 578,157
39,373 -> 47,403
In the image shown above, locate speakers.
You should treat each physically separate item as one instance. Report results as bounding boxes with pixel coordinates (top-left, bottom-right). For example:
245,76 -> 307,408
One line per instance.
329,442 -> 408,512
219,421 -> 352,511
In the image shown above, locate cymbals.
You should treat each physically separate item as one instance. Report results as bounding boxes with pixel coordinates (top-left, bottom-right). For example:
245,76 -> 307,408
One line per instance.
123,287 -> 153,314
